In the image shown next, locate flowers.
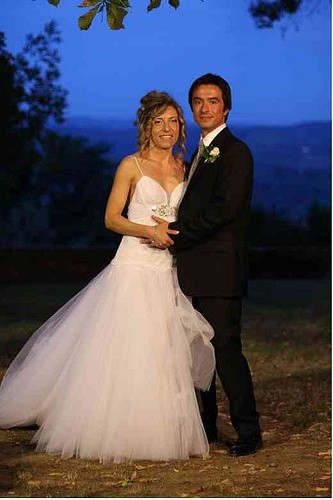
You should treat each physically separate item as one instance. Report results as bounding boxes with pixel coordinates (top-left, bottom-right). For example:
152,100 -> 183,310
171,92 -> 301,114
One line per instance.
201,146 -> 220,165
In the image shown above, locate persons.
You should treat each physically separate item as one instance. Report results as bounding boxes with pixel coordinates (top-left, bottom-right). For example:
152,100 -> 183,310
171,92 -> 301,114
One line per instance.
1,90 -> 216,460
139,72 -> 263,456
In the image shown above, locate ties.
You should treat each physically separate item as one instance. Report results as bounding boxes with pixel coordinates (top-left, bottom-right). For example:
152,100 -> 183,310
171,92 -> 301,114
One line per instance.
177,140 -> 205,208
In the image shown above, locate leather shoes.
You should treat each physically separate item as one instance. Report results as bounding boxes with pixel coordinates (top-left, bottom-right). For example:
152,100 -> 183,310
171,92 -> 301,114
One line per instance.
230,433 -> 262,456
207,428 -> 219,444
